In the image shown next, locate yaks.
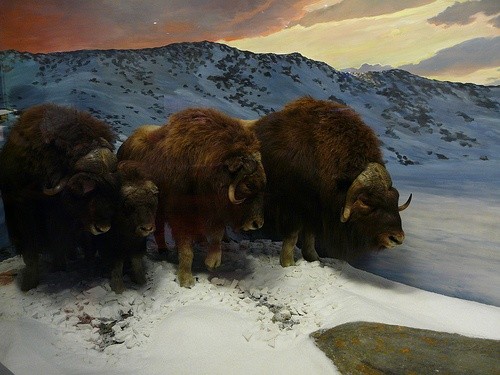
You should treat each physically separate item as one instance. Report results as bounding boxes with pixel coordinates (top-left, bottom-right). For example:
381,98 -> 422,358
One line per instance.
116,108 -> 267,289
0,104 -> 122,292
249,96 -> 412,268
102,158 -> 161,294
